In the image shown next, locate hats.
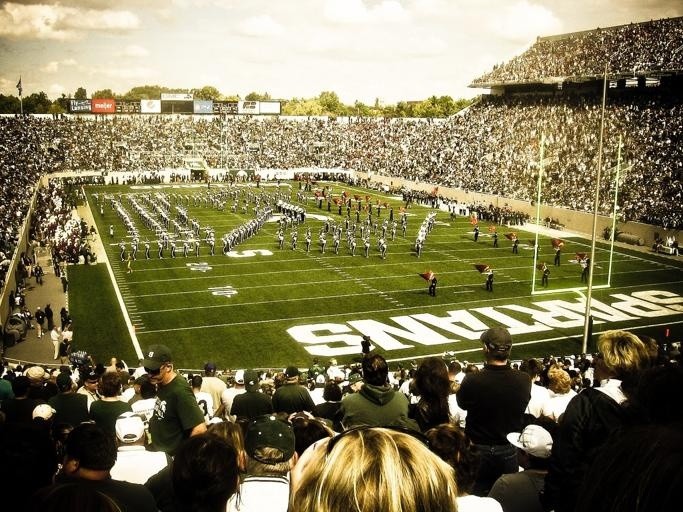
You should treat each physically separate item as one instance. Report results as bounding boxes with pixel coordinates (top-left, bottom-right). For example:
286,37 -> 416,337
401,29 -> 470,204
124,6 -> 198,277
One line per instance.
114,410 -> 146,444
30,403 -> 56,423
27,361 -> 100,394
202,362 -> 216,374
284,366 -> 303,377
504,422 -> 553,460
243,417 -> 296,465
234,368 -> 259,392
479,326 -> 513,351
141,344 -> 172,371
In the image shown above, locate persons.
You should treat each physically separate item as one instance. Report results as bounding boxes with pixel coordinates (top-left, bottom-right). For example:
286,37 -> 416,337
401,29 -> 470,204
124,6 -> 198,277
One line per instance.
578,254 -> 589,283
467,16 -> 683,85
540,262 -> 548,288
0,323 -> 682,512
427,270 -> 436,296
1,102 -> 682,262
8,263 -> 70,361
482,266 -> 493,291
